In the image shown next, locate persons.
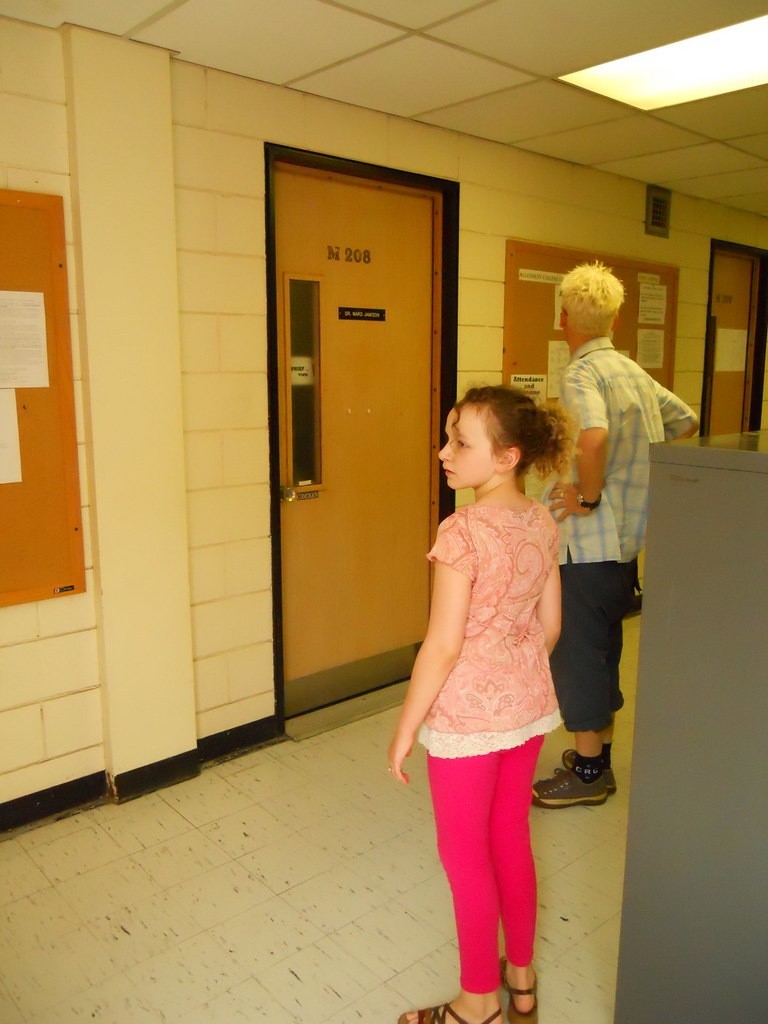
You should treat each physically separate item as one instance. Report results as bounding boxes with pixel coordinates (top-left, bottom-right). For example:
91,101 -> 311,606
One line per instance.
529,261 -> 701,811
386,382 -> 565,1024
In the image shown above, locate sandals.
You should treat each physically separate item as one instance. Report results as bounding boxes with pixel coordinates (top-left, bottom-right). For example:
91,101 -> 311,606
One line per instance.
497,951 -> 539,1024
395,1004 -> 504,1024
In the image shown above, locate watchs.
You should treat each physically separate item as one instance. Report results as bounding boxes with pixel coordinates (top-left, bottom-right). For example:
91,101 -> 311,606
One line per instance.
577,488 -> 604,509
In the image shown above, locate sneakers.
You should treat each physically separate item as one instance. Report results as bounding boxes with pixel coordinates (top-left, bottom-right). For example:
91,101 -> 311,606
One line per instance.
532,770 -> 607,808
562,748 -> 618,796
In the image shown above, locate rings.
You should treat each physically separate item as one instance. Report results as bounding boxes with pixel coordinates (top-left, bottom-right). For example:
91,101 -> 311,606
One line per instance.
388,768 -> 392,772
561,492 -> 565,498
